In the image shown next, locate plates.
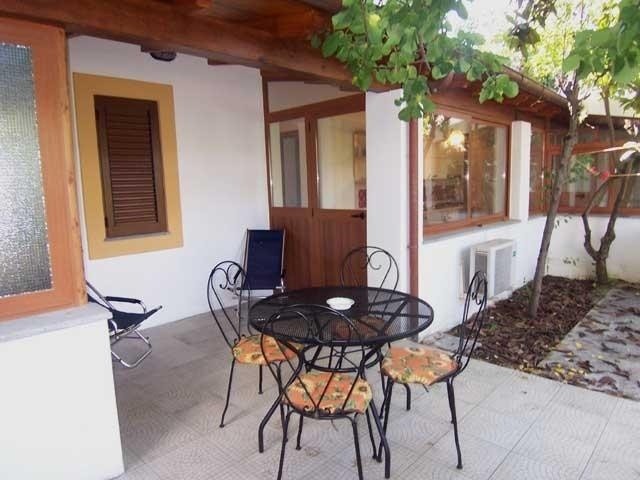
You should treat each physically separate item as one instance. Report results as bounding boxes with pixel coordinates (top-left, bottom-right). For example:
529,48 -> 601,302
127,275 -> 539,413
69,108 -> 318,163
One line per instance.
325,297 -> 355,309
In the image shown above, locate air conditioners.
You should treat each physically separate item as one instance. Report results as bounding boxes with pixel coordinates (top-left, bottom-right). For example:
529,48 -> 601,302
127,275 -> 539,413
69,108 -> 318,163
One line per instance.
468,238 -> 518,300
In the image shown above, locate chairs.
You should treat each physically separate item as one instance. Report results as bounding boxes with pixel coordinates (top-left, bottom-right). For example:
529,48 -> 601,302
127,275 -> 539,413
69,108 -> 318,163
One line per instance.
84,282 -> 161,369
260,303 -> 377,479
207,262 -> 304,442
328,246 -> 411,411
227,229 -> 286,320
378,271 -> 488,469
